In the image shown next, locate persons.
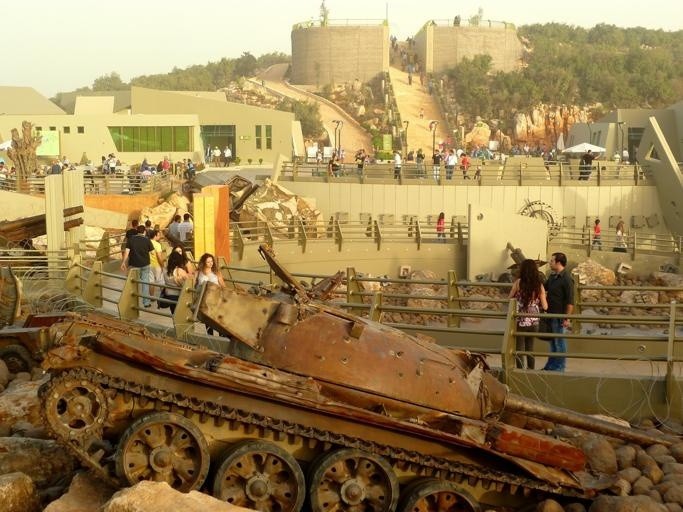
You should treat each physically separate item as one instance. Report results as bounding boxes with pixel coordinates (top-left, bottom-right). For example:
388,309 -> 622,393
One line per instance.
393,151 -> 402,180
138,156 -> 169,179
121,213 -> 224,337
51,155 -> 69,175
420,108 -> 424,119
432,150 -> 471,180
616,221 -> 628,252
507,259 -> 548,369
101,153 -> 117,175
214,145 -> 232,167
614,148 -> 628,164
183,158 -> 194,172
579,150 -> 601,180
541,253 -> 574,371
390,36 -> 434,96
316,148 -> 366,177
311,17 -> 314,26
320,15 -> 324,27
437,213 -> 446,237
1,162 -> 16,188
429,122 -> 433,131
408,148 -> 425,164
592,220 -> 603,251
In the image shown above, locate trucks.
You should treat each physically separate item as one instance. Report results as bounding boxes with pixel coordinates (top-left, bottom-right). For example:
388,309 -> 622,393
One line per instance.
182,170 -> 323,241
1,264 -> 65,372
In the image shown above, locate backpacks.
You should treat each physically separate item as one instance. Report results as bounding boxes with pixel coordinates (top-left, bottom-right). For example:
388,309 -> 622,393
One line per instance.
519,303 -> 540,327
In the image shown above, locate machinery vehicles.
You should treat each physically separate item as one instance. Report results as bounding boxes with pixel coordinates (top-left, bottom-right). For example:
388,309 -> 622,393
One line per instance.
38,243 -> 678,512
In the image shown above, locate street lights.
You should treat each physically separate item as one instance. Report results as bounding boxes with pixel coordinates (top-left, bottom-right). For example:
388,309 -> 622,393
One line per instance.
618,121 -> 626,162
430,120 -> 439,156
332,119 -> 344,160
403,120 -> 409,156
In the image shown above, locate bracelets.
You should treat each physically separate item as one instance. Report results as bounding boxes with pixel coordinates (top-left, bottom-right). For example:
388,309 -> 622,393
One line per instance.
565,319 -> 571,322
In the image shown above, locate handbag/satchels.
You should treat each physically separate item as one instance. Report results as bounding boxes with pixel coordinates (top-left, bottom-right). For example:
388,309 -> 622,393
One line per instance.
157,289 -> 169,308
536,320 -> 552,341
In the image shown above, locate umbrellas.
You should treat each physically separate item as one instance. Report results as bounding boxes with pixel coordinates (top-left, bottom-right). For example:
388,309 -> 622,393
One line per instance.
557,132 -> 564,151
